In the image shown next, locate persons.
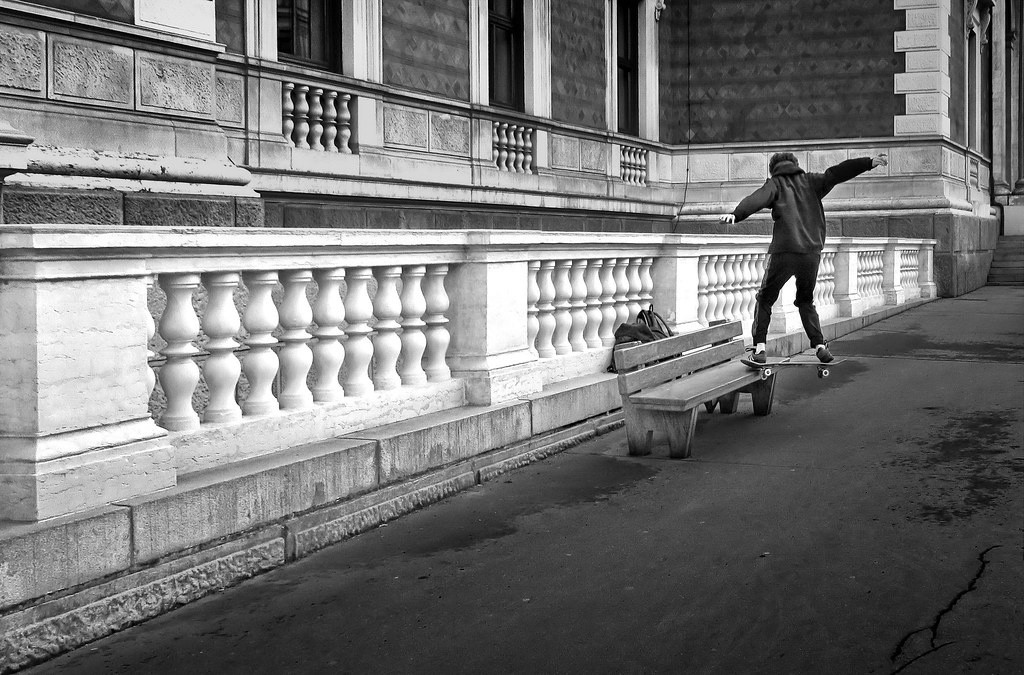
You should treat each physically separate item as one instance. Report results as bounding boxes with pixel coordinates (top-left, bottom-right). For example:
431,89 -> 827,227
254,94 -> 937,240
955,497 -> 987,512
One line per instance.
719,153 -> 889,362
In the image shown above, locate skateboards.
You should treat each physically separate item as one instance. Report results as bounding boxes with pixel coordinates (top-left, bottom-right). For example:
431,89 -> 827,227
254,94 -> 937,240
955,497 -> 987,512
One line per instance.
740,357 -> 848,382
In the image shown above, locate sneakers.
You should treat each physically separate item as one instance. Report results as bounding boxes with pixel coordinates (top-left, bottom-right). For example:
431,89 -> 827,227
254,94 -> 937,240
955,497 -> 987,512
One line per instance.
744,347 -> 767,363
816,339 -> 835,363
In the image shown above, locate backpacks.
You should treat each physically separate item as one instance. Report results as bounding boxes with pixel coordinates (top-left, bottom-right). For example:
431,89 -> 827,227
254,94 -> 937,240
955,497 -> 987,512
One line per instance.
636,304 -> 683,359
607,316 -> 661,374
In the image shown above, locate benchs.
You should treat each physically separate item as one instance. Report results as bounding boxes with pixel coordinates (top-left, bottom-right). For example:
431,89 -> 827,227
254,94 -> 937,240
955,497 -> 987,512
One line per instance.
614,318 -> 791,458
